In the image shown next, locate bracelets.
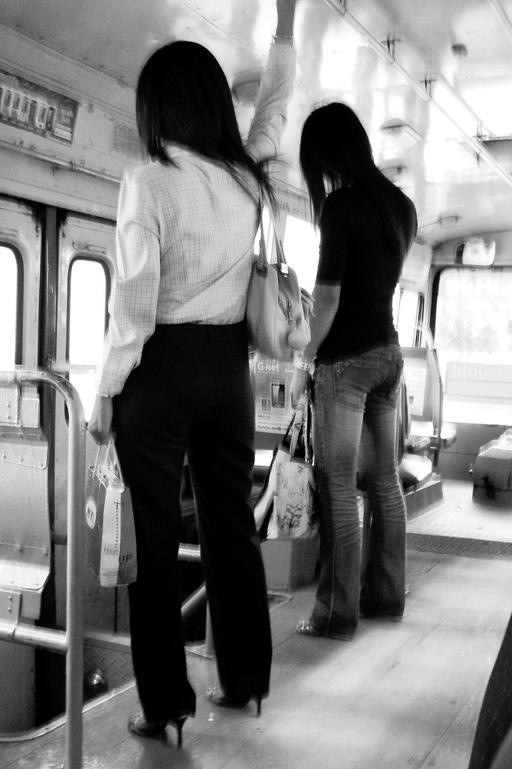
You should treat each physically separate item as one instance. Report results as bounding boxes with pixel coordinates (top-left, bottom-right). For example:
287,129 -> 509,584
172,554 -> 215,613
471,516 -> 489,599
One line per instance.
272,34 -> 292,41
294,359 -> 313,372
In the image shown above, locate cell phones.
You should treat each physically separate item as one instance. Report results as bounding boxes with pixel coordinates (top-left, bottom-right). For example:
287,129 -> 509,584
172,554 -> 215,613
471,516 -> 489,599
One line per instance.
270,382 -> 286,414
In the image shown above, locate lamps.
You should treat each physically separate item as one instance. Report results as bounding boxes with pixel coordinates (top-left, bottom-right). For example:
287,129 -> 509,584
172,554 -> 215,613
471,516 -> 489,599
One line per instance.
383,125 -> 420,149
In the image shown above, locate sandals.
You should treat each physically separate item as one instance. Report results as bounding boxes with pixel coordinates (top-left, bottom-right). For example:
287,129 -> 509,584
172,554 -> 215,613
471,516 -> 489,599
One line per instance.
296,619 -> 328,635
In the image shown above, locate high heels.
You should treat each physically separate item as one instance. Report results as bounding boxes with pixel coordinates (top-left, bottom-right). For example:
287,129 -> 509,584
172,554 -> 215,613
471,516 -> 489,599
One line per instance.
205,678 -> 269,717
129,707 -> 187,747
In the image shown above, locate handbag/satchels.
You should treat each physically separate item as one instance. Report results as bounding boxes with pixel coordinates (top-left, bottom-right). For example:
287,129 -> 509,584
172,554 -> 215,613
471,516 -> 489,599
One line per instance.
246,191 -> 316,362
251,392 -> 321,543
84,437 -> 138,588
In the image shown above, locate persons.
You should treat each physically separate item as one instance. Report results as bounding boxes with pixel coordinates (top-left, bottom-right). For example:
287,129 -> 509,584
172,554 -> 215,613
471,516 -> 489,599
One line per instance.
288,103 -> 420,641
87,0 -> 296,750
